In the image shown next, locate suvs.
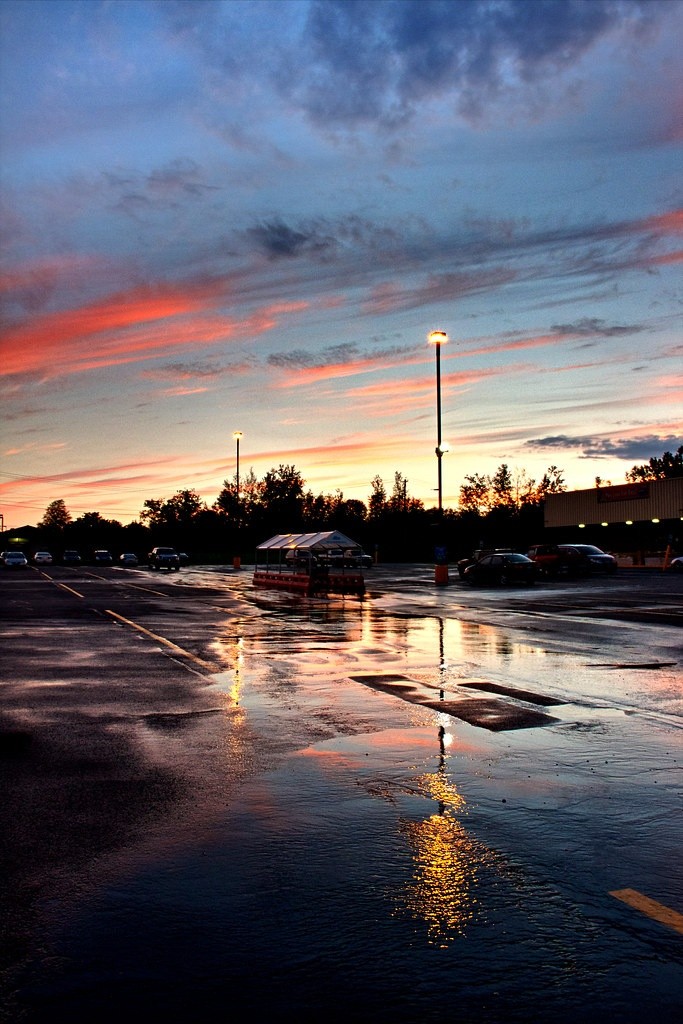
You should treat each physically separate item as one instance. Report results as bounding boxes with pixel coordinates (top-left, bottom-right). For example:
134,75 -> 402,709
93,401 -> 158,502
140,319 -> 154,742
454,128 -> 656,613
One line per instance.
90,549 -> 113,565
456,548 -> 519,577
62,549 -> 80,565
147,547 -> 180,571
285,550 -> 318,568
343,549 -> 374,569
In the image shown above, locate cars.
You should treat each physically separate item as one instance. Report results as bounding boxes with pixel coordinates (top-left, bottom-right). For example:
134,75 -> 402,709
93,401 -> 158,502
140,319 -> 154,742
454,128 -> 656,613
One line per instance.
4,552 -> 27,567
117,554 -> 139,566
32,551 -> 54,564
0,551 -> 10,563
524,544 -> 569,581
556,544 -> 619,576
177,553 -> 190,565
463,552 -> 540,587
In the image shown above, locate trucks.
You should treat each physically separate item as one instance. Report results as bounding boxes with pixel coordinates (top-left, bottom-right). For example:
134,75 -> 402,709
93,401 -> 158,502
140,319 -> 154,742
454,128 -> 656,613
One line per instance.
315,548 -> 344,567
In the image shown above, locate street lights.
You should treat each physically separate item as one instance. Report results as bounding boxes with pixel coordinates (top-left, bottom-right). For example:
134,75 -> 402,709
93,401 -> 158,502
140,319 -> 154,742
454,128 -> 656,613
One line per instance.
425,332 -> 452,564
235,431 -> 243,561
